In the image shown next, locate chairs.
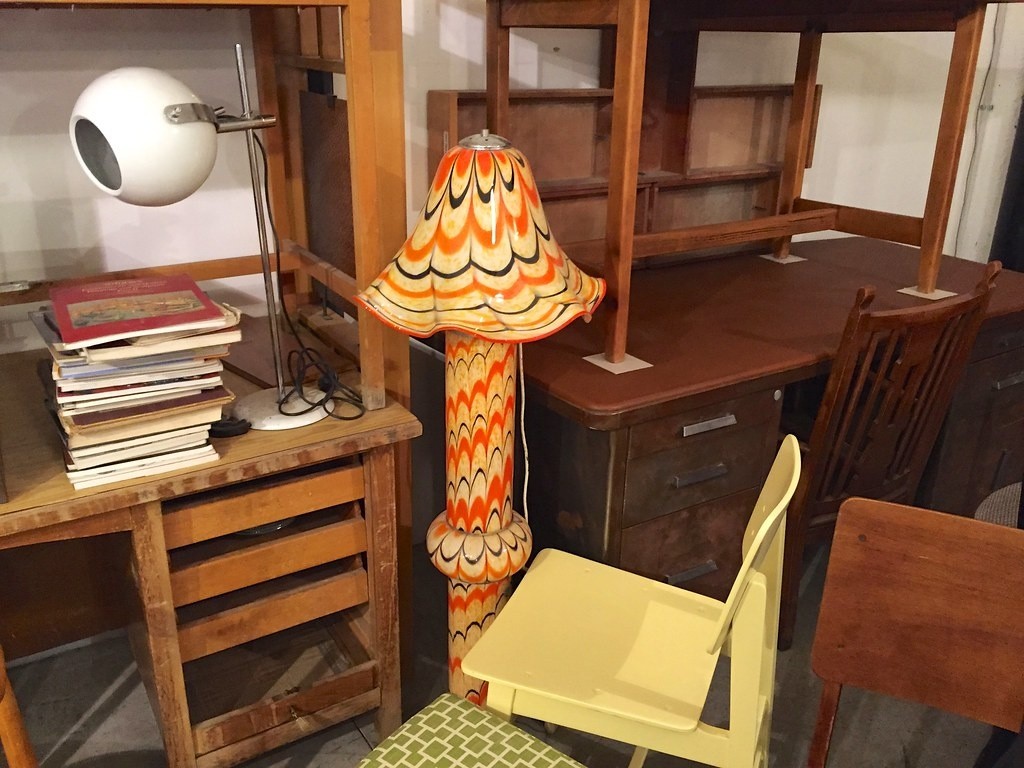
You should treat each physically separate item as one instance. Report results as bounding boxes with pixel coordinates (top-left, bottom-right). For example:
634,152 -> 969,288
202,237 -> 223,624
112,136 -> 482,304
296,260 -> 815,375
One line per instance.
462,433 -> 802,768
779,260 -> 1003,647
806,497 -> 1024,768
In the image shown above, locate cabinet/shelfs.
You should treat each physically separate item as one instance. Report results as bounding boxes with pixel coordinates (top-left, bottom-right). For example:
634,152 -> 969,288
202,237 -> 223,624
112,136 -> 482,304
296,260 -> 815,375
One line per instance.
484,0 -> 1024,576
0,0 -> 424,768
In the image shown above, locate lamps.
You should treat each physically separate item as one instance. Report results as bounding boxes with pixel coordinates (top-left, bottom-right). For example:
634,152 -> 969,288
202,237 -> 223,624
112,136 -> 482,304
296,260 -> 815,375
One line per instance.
71,41 -> 335,430
351,129 -> 607,706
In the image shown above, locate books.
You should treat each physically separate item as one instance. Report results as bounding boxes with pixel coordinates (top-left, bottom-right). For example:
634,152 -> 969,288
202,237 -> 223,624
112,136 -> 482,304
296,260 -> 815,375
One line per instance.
28,273 -> 241,490
